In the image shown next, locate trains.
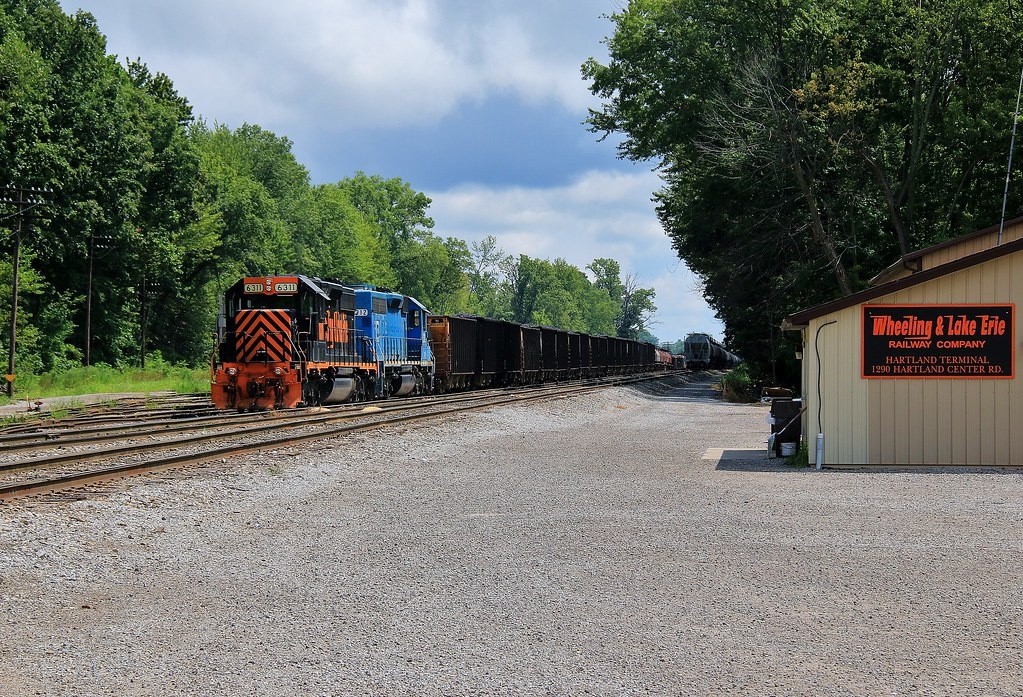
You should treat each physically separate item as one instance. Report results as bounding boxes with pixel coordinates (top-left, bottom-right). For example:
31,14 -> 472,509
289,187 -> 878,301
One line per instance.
683,331 -> 744,370
208,273 -> 684,410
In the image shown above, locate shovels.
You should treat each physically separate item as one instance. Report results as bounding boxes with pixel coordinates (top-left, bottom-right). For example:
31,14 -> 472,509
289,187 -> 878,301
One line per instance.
767,407 -> 808,458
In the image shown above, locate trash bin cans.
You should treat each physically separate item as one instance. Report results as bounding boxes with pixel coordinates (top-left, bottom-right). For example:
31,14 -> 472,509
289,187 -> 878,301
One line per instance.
773,400 -> 801,458
